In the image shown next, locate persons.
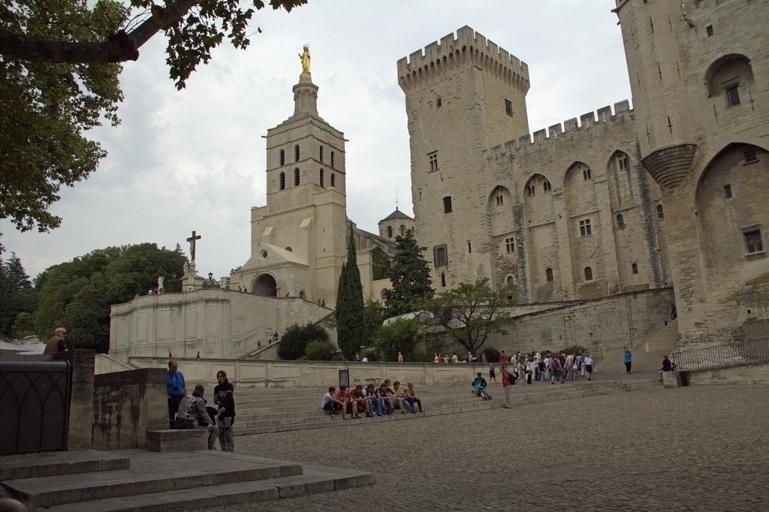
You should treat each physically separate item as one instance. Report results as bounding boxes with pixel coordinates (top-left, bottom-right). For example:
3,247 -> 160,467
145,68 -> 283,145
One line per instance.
362,356 -> 368,363
318,296 -> 325,306
624,346 -> 632,375
300,289 -> 305,299
500,351 -> 509,376
488,362 -> 497,384
44,328 -> 66,356
134,286 -> 160,297
165,361 -> 235,451
663,355 -> 682,386
472,372 -> 487,397
238,286 -> 247,293
501,366 -> 511,407
355,353 -> 359,361
321,379 -> 423,420
257,332 -> 278,350
398,352 -> 404,363
299,47 -> 310,73
433,351 -> 487,364
510,352 -> 594,384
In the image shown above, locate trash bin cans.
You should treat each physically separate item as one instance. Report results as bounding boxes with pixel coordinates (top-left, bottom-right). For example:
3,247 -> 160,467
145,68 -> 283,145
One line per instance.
662,371 -> 676,388
676,368 -> 689,386
